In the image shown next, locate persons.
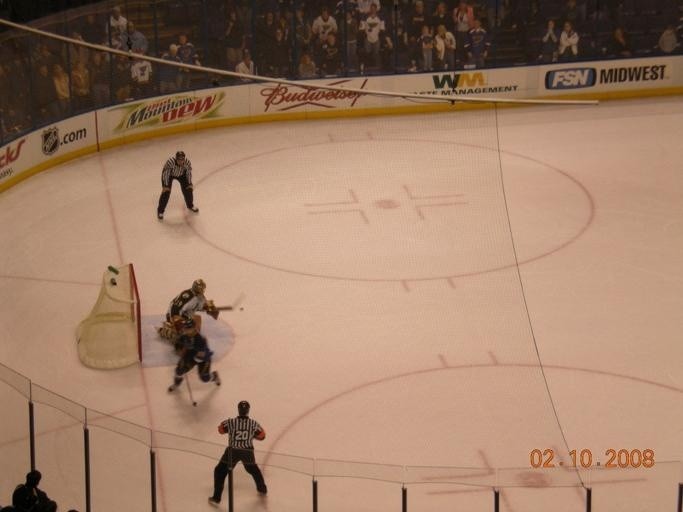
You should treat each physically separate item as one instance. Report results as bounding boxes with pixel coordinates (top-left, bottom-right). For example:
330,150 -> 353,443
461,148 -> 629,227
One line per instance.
157,151 -> 200,220
206,398 -> 268,506
157,278 -> 219,345
168,319 -> 220,393
1,1 -> 681,149
10,469 -> 57,512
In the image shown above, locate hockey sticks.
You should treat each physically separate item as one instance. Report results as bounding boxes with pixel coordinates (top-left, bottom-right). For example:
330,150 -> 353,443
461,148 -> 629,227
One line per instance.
210,291 -> 242,312
184,373 -> 197,407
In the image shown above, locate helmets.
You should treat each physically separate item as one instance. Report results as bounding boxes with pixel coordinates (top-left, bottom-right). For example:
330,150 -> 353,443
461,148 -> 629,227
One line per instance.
175,151 -> 185,160
238,401 -> 250,409
192,279 -> 207,295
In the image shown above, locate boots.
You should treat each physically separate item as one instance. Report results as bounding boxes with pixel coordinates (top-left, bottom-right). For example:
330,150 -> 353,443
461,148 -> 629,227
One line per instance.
168,383 -> 180,392
212,371 -> 220,386
158,212 -> 163,219
208,496 -> 221,504
189,206 -> 199,212
